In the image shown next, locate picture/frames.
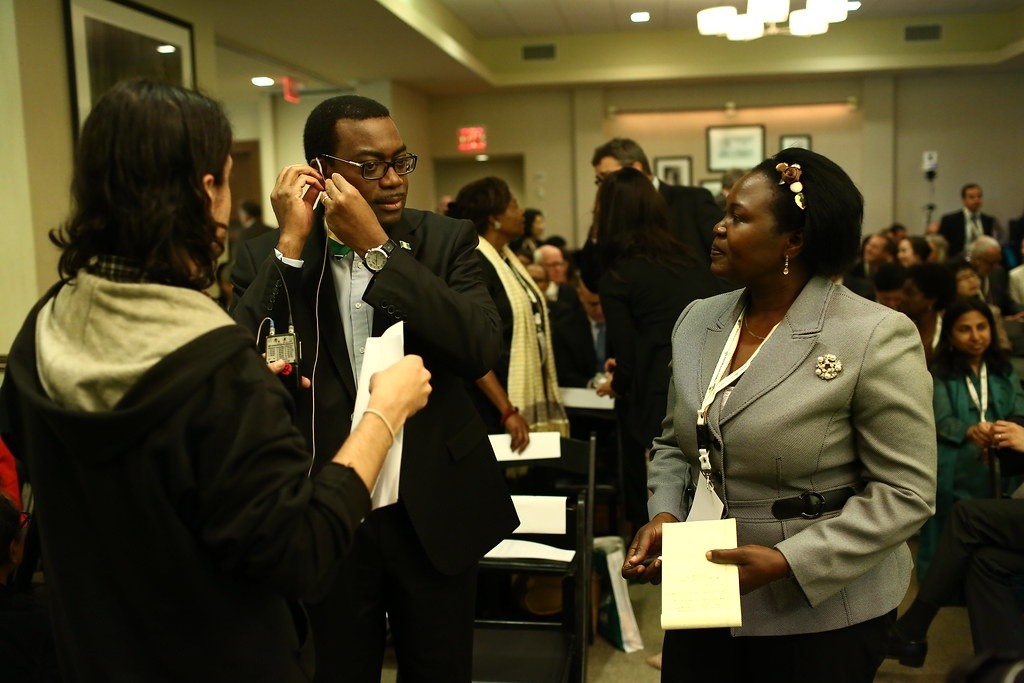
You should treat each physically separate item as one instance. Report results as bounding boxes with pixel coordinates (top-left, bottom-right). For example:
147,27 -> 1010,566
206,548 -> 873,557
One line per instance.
779,133 -> 812,152
704,123 -> 766,173
62,0 -> 196,155
652,155 -> 693,188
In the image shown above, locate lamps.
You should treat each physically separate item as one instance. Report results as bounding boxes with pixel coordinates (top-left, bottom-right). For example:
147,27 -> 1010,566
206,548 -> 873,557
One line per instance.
696,0 -> 863,43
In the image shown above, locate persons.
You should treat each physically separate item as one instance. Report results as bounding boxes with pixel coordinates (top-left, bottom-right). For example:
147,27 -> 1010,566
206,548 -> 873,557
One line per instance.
885,418 -> 1024,668
859,182 -> 1024,353
925,299 -> 1024,608
213,198 -> 278,309
443,135 -> 731,529
229,94 -> 502,683
621,148 -> 937,683
1,73 -> 433,680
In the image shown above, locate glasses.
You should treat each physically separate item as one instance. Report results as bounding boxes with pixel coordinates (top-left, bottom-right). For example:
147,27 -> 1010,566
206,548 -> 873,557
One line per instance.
321,151 -> 417,180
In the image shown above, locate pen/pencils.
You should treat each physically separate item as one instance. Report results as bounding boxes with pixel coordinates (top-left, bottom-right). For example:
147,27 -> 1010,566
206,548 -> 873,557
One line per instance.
624,556 -> 662,569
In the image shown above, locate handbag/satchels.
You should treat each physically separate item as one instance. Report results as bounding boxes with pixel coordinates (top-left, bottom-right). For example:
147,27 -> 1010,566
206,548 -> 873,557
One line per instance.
593,536 -> 645,655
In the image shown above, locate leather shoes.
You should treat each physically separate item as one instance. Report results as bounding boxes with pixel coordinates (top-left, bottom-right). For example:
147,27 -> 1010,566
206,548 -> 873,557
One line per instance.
887,618 -> 928,668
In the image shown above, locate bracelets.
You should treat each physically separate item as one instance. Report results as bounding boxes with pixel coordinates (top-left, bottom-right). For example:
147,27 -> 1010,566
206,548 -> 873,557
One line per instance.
502,406 -> 518,423
363,409 -> 395,451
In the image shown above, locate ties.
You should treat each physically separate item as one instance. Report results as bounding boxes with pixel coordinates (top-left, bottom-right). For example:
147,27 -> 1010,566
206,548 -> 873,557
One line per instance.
971,215 -> 979,240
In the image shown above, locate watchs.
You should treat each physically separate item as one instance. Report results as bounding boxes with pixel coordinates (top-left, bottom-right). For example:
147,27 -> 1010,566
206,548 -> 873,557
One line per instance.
362,239 -> 396,274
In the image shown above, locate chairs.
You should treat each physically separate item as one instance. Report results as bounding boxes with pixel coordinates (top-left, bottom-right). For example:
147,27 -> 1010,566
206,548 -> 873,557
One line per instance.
468,434 -> 598,682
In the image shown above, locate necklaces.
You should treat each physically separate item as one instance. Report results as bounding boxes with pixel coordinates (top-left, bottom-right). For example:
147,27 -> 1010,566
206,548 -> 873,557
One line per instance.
744,312 -> 765,342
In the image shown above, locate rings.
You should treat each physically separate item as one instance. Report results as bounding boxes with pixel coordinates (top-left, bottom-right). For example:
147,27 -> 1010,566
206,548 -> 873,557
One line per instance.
999,433 -> 1002,439
